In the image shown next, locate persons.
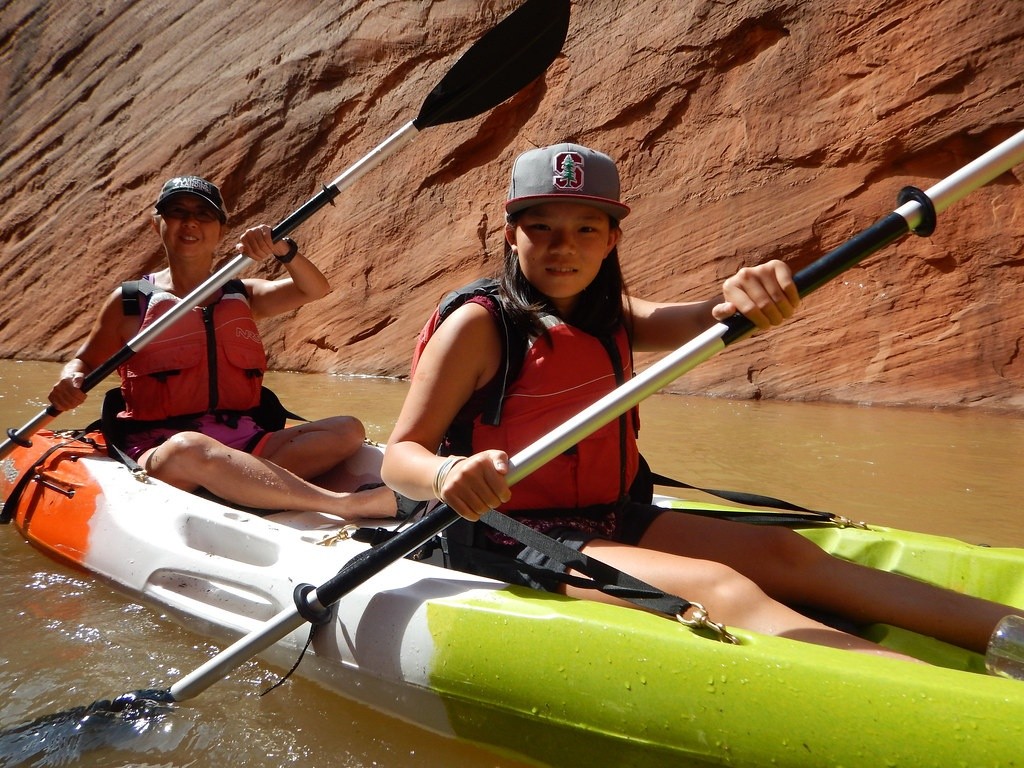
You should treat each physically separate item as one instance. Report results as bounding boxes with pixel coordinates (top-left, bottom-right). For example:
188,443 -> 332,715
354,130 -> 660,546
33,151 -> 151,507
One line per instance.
381,142 -> 1021,671
47,176 -> 427,523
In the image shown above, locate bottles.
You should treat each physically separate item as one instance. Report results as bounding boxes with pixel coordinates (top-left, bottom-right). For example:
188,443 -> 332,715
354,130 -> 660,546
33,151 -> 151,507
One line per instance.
984,614 -> 1024,681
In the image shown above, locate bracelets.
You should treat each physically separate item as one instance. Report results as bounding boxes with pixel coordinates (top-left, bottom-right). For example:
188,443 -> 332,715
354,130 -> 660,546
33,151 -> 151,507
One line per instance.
273,237 -> 299,263
433,454 -> 464,503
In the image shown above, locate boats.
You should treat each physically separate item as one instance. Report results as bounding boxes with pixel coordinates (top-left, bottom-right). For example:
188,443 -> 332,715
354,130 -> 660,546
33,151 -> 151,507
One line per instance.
1,425 -> 1024,768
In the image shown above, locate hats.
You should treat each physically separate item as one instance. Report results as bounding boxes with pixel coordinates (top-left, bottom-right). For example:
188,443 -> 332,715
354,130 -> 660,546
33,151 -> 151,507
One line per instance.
155,176 -> 229,226
502,142 -> 630,221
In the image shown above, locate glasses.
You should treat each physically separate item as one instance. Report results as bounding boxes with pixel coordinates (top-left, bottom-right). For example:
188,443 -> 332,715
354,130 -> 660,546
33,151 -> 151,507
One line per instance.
162,204 -> 222,223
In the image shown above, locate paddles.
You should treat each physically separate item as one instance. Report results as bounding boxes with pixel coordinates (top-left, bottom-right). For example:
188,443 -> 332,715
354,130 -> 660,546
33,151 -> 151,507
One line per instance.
0,128 -> 1024,768
0,0 -> 572,463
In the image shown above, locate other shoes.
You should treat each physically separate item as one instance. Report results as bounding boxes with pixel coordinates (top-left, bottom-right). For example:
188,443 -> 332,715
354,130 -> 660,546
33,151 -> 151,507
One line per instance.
356,480 -> 426,523
